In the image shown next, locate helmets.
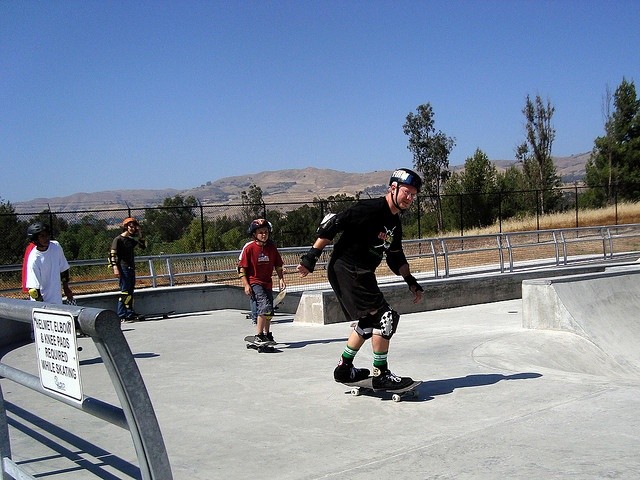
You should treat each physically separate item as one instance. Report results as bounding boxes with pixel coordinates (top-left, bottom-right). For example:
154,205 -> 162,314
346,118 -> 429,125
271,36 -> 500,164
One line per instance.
123,218 -> 137,228
389,169 -> 422,194
26,222 -> 49,242
246,219 -> 271,238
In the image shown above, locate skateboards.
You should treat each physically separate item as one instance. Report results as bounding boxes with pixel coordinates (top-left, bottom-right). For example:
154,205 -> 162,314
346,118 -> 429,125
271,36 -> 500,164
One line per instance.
272,290 -> 287,309
344,376 -> 423,402
136,311 -> 175,321
241,313 -> 252,319
245,336 -> 276,353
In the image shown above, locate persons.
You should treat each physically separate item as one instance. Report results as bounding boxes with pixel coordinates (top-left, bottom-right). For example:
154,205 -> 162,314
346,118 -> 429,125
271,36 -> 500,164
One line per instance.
108,217 -> 145,321
25,222 -> 73,306
296,167 -> 423,394
236,218 -> 286,345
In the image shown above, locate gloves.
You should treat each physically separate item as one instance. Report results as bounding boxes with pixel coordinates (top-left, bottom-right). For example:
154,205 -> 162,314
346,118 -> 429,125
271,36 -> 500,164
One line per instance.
403,275 -> 424,294
301,248 -> 321,273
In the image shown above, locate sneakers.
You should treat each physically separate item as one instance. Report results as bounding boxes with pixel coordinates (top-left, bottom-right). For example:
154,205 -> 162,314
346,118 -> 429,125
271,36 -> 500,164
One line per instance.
372,365 -> 414,394
253,333 -> 268,345
120,315 -> 133,323
334,357 -> 370,383
131,313 -> 141,320
263,331 -> 277,345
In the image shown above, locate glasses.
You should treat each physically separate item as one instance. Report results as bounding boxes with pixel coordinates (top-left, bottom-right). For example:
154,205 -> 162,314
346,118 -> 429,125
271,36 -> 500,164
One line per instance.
399,187 -> 418,200
